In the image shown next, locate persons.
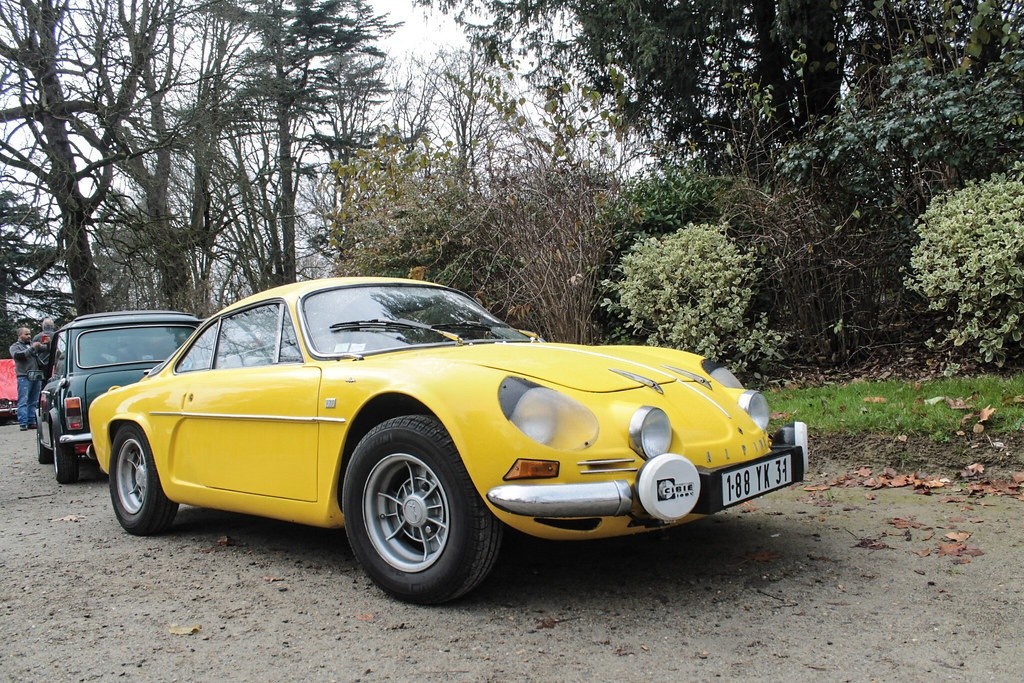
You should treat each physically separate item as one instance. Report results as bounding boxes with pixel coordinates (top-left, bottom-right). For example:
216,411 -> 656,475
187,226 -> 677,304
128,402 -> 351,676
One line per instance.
9,318 -> 56,430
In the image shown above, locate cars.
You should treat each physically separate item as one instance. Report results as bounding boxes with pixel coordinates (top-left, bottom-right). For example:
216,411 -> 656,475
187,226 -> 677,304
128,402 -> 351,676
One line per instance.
0,358 -> 19,424
86,275 -> 812,609
26,308 -> 206,485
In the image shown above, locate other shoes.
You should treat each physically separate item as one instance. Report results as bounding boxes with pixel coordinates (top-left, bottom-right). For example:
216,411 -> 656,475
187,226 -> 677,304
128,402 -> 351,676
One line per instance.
19,424 -> 27,431
28,423 -> 37,429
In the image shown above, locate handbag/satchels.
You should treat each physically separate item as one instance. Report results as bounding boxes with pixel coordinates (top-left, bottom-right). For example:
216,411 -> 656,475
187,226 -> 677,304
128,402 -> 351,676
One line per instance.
27,370 -> 45,382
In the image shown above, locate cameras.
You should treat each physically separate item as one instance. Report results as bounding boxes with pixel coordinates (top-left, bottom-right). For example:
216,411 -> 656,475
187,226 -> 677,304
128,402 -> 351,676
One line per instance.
41,335 -> 47,343
